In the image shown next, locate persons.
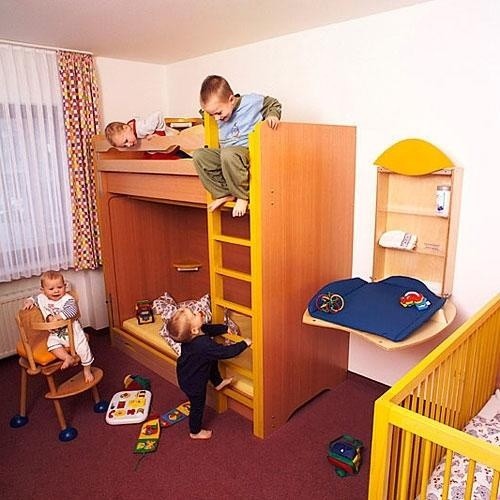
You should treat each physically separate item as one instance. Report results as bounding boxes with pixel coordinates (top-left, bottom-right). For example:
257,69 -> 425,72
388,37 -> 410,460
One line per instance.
167,307 -> 252,439
104,110 -> 180,148
22,270 -> 95,383
193,75 -> 282,218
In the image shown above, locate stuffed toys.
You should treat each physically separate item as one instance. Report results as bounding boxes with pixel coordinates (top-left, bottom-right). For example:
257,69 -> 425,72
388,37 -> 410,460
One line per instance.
135,299 -> 154,323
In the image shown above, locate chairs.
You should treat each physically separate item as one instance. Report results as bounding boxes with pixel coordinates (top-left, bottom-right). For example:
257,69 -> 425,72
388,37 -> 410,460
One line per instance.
16,295 -> 104,432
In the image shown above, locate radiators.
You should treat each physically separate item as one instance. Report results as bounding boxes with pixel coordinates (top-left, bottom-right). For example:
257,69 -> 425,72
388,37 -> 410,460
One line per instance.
0,280 -> 71,360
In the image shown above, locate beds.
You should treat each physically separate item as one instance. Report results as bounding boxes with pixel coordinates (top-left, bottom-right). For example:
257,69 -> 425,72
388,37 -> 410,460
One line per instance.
90,121 -> 356,439
368,294 -> 500,500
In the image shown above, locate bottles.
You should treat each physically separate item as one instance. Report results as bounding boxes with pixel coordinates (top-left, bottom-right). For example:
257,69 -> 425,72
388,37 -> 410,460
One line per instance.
435,185 -> 452,218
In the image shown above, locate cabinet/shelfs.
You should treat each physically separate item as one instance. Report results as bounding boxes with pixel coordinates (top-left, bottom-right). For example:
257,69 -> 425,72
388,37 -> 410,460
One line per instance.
372,166 -> 463,298
